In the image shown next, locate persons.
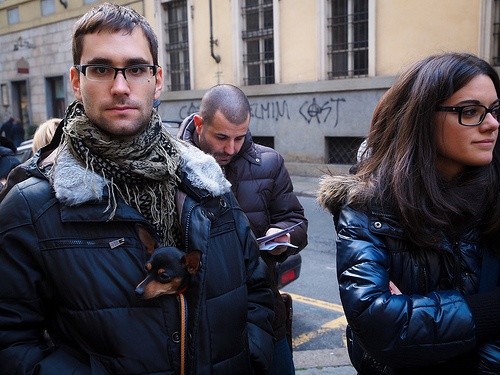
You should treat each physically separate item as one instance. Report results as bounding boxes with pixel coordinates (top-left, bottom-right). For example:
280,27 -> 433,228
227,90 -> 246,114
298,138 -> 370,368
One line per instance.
0,2 -> 277,375
177,84 -> 309,375
320,53 -> 500,375
0,117 -> 64,181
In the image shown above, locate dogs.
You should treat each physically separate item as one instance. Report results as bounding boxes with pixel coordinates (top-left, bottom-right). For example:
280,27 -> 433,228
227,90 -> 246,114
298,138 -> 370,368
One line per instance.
133,224 -> 204,301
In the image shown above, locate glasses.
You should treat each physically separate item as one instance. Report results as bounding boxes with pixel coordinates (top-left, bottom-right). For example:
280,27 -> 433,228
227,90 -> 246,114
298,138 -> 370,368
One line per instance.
73,64 -> 159,84
435,104 -> 500,127
